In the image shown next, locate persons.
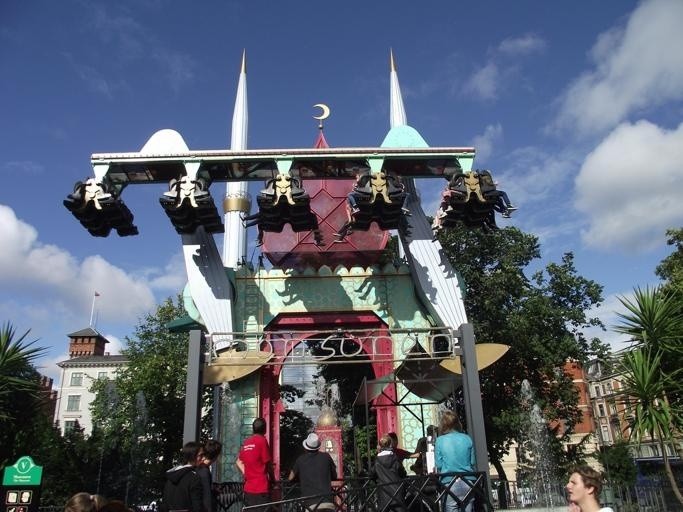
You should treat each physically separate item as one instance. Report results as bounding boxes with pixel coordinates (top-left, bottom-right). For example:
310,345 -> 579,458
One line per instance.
236,417 -> 276,512
64,492 -> 103,512
288,433 -> 337,512
368,411 -> 477,512
97,500 -> 133,512
161,442 -> 206,512
566,465 -> 614,512
194,440 -> 222,512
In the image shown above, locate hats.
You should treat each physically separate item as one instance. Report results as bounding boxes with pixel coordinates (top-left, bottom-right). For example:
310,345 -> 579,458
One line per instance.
302,433 -> 321,451
